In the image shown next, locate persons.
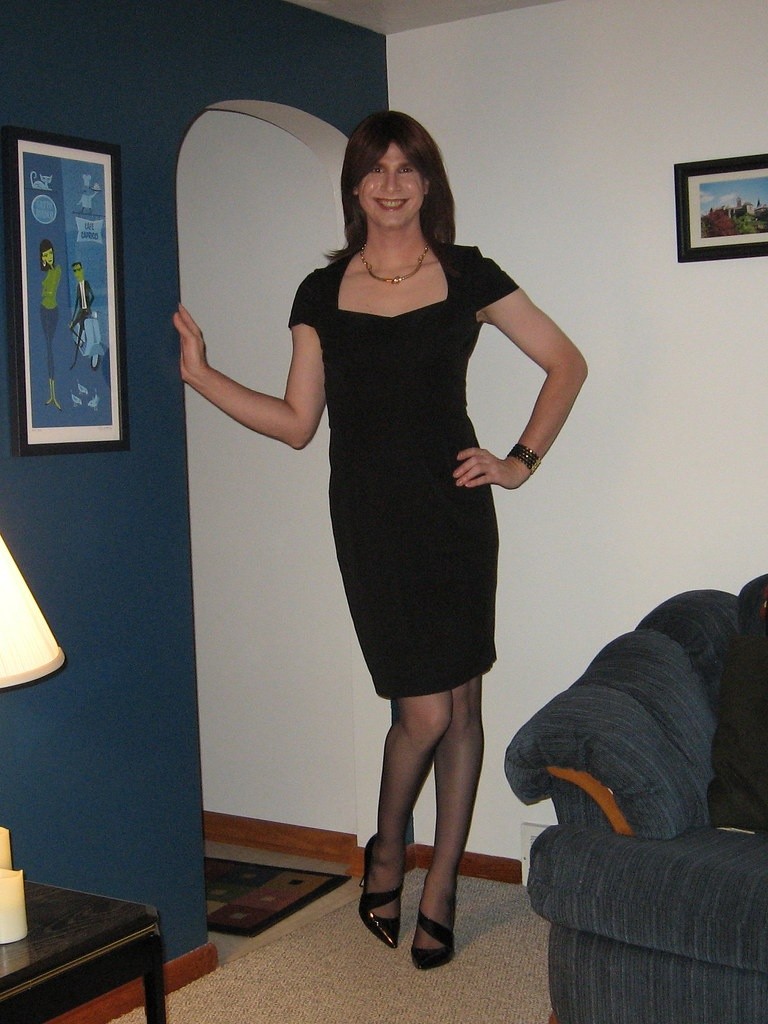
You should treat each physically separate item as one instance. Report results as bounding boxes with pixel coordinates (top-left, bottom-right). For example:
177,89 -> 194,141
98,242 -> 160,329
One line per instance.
173,110 -> 588,971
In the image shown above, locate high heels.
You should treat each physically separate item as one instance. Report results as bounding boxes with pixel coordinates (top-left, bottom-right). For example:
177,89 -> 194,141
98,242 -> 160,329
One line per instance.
411,901 -> 456,969
360,833 -> 403,948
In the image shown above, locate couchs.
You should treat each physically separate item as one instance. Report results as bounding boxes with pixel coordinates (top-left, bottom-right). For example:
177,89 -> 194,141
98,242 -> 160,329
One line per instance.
504,574 -> 768,1024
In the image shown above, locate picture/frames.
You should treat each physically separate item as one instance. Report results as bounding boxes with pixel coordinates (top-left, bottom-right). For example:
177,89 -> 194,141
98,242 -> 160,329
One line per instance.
674,153 -> 768,263
0,127 -> 131,459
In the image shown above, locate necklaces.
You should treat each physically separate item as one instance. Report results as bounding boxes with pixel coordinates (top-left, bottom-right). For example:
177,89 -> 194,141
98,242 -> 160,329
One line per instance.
345,242 -> 441,286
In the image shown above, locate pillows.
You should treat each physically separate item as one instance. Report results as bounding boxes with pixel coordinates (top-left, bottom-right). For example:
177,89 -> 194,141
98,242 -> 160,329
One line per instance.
706,573 -> 768,835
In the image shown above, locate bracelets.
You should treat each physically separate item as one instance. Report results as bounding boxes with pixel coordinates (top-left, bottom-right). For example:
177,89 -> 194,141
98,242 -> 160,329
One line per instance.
501,442 -> 543,476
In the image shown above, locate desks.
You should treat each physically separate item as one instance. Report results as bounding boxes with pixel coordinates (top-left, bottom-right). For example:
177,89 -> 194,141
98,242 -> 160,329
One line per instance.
0,881 -> 167,1024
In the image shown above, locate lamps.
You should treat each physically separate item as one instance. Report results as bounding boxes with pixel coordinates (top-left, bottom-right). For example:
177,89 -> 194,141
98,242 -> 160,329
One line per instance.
0,535 -> 67,946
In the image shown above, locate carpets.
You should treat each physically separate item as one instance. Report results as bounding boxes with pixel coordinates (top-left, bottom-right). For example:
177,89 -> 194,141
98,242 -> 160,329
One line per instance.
204,854 -> 353,938
